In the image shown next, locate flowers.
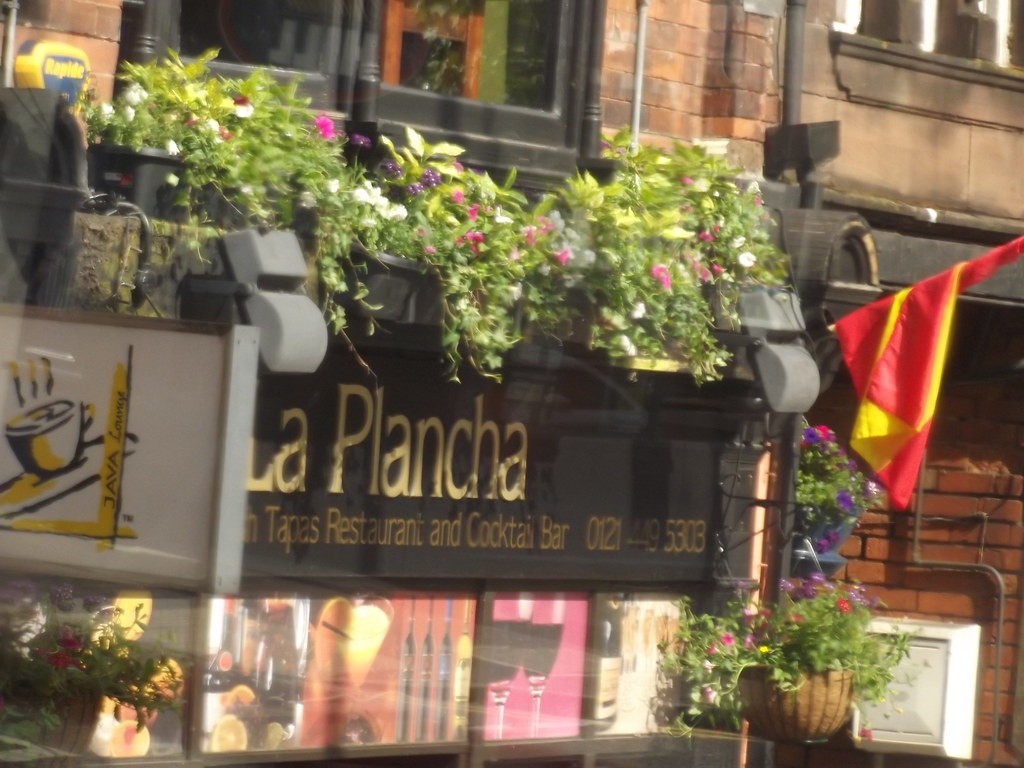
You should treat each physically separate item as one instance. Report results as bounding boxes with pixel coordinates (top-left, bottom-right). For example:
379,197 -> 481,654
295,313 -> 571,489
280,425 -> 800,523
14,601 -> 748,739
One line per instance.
654,571 -> 926,737
24,576 -> 180,721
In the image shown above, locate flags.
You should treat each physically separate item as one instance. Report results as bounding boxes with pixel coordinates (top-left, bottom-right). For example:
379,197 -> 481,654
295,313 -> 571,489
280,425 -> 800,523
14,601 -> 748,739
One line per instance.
837,236 -> 1023,511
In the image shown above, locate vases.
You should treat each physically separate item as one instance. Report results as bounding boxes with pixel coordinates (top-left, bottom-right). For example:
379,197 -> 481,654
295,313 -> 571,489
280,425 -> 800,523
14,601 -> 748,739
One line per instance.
735,668 -> 856,744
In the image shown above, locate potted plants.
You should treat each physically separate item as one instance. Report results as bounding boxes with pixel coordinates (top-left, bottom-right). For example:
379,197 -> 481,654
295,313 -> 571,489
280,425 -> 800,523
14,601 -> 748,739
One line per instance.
78,42 -> 789,386
794,422 -> 886,566
